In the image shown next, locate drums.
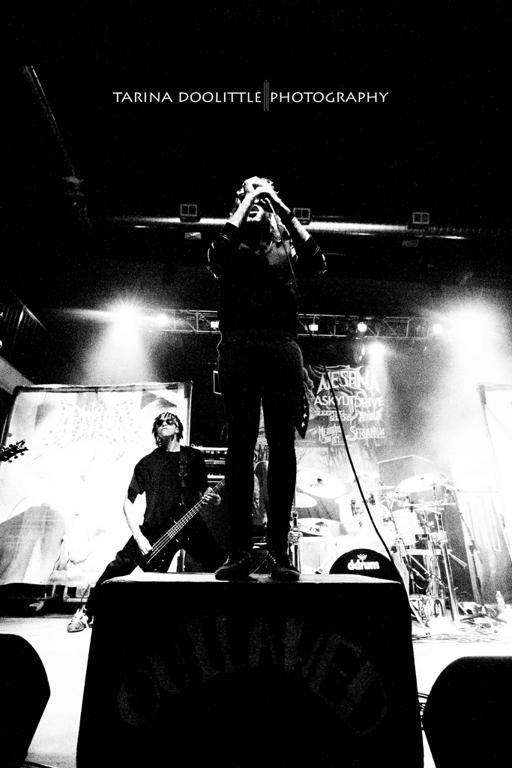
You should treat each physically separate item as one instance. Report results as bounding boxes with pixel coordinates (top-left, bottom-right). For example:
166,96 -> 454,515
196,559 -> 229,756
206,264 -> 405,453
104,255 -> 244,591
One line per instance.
391,509 -> 424,544
330,548 -> 402,582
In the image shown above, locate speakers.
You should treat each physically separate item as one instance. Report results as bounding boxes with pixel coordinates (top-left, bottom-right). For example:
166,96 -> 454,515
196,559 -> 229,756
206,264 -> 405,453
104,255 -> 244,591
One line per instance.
74,571 -> 424,768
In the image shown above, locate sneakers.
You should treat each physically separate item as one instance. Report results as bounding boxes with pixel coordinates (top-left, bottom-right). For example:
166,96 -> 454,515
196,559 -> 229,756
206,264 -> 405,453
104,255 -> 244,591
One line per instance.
66,606 -> 90,632
211,551 -> 305,582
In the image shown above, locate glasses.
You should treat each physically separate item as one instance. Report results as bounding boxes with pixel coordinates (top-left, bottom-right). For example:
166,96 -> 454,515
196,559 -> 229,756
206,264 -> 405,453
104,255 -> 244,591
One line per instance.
154,418 -> 179,429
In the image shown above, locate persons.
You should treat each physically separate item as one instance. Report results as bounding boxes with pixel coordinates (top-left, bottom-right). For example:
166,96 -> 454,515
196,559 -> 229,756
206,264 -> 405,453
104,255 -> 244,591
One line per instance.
205,174 -> 328,582
66,413 -> 221,633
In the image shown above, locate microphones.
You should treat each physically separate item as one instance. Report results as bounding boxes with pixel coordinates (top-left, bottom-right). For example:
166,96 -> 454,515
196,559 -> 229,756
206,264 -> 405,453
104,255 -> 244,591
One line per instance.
254,183 -> 271,204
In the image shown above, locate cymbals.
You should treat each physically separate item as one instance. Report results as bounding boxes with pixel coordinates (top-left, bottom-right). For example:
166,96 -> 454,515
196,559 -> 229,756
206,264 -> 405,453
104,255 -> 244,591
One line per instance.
290,517 -> 340,536
295,470 -> 346,499
294,492 -> 317,509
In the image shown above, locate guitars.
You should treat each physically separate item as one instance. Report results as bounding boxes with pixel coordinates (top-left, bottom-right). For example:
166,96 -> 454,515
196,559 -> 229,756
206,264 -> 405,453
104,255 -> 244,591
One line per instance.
120,479 -> 224,574
